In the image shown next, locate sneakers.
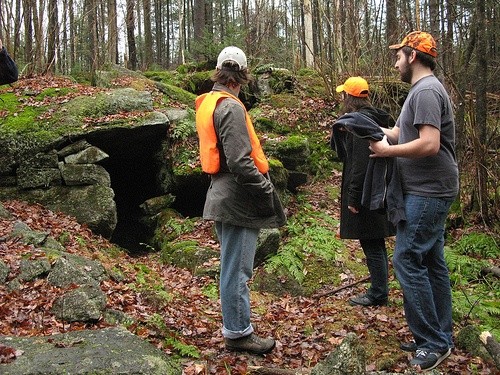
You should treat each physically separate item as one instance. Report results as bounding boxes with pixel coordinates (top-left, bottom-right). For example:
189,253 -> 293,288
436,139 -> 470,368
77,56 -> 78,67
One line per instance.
348,293 -> 384,307
409,345 -> 452,373
401,340 -> 418,351
225,333 -> 276,353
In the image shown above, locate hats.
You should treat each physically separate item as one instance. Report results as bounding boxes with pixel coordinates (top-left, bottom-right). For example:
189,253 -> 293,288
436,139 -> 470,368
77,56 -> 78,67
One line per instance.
388,30 -> 437,57
217,45 -> 248,70
335,76 -> 369,97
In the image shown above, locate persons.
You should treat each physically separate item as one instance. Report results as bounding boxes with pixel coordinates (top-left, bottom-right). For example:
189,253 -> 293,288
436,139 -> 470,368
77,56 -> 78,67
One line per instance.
197,45 -> 277,353
334,76 -> 398,304
365,30 -> 461,371
0,41 -> 19,85
